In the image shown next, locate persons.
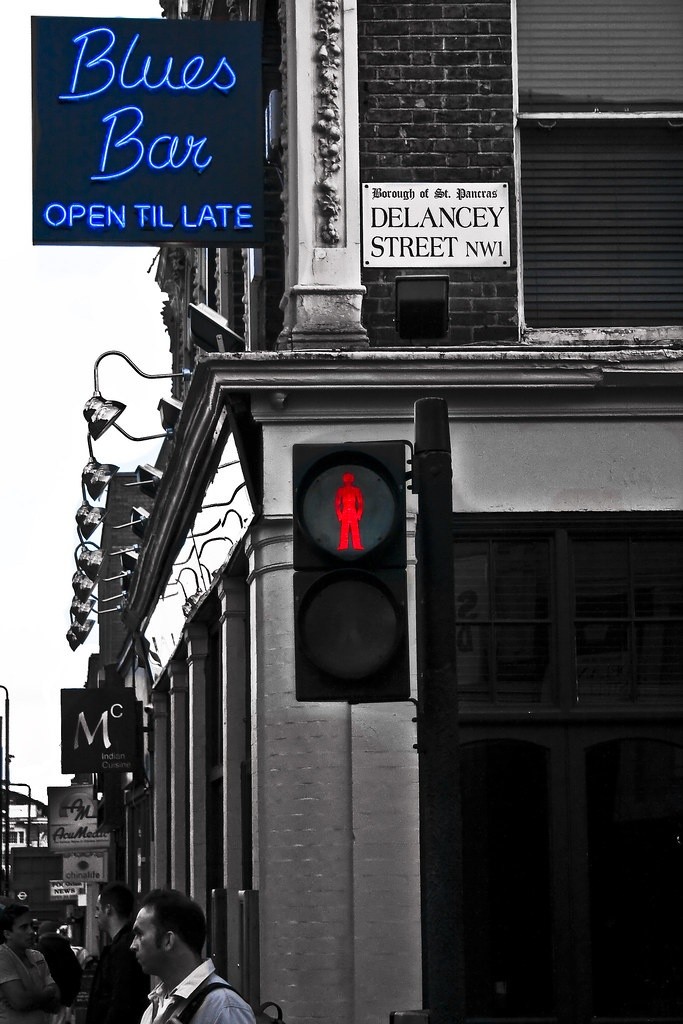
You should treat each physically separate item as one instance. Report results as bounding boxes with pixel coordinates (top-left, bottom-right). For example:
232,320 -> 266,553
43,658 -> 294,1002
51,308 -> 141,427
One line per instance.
129,888 -> 256,1024
0,903 -> 84,1024
85,882 -> 150,1024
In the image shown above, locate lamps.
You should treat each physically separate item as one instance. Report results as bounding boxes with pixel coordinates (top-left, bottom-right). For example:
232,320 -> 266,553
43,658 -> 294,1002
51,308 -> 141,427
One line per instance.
395,275 -> 450,338
66,351 -> 191,652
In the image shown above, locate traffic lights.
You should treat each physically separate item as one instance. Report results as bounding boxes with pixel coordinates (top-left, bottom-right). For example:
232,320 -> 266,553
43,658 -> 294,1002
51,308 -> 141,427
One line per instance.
296,451 -> 403,564
295,570 -> 408,706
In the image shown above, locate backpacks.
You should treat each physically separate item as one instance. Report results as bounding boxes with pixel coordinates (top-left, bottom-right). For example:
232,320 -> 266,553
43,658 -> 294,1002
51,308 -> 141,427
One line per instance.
166,982 -> 286,1024
